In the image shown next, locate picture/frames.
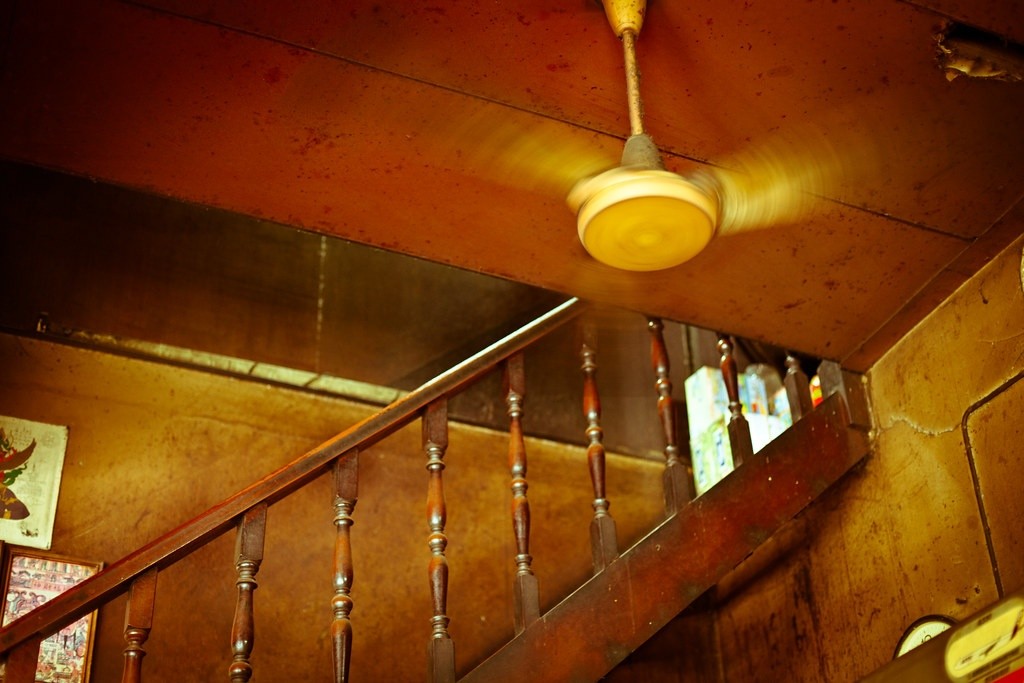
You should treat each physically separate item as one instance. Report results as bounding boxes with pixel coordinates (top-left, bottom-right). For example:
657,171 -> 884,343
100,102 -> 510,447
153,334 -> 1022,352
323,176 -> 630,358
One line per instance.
0,547 -> 107,683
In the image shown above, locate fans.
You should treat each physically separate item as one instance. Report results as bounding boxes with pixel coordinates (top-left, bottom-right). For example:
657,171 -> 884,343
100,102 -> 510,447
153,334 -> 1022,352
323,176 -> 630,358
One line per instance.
421,1 -> 868,322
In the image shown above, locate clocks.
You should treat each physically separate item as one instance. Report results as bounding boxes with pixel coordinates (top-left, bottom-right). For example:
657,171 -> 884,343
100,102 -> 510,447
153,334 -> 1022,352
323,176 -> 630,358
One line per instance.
893,614 -> 956,661
